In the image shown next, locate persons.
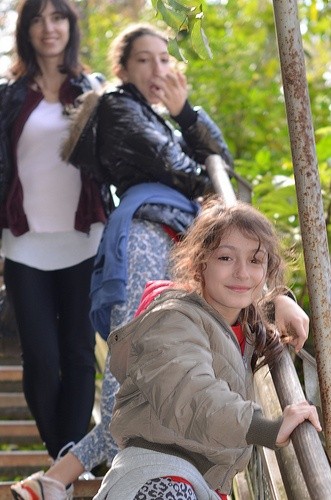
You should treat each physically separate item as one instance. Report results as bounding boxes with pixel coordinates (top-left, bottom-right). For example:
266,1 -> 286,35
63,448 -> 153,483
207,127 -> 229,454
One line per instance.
0,1 -> 114,482
10,19 -> 235,500
92,200 -> 323,500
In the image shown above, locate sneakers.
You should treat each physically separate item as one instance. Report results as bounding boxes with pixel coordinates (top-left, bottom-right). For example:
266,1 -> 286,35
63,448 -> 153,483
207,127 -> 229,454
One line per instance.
10,471 -> 76,500
